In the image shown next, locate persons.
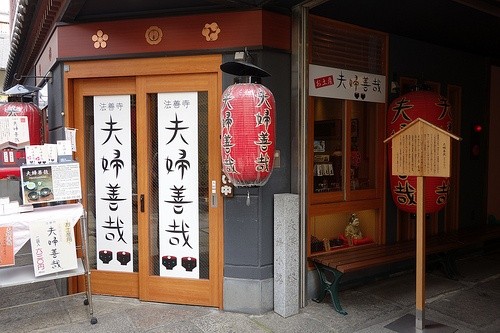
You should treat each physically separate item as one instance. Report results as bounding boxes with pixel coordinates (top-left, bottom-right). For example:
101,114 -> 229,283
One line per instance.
345,212 -> 363,239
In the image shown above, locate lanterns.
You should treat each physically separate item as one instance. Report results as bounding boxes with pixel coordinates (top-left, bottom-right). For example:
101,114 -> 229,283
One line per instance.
388,89 -> 458,218
219,82 -> 276,188
352,148 -> 361,167
0,96 -> 43,145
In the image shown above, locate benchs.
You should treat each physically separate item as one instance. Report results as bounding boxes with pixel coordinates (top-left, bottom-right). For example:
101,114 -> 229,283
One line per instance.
425,224 -> 500,279
308,239 -> 415,313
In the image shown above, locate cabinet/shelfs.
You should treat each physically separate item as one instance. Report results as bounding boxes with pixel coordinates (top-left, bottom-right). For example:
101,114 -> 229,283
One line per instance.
0,181 -> 94,322
